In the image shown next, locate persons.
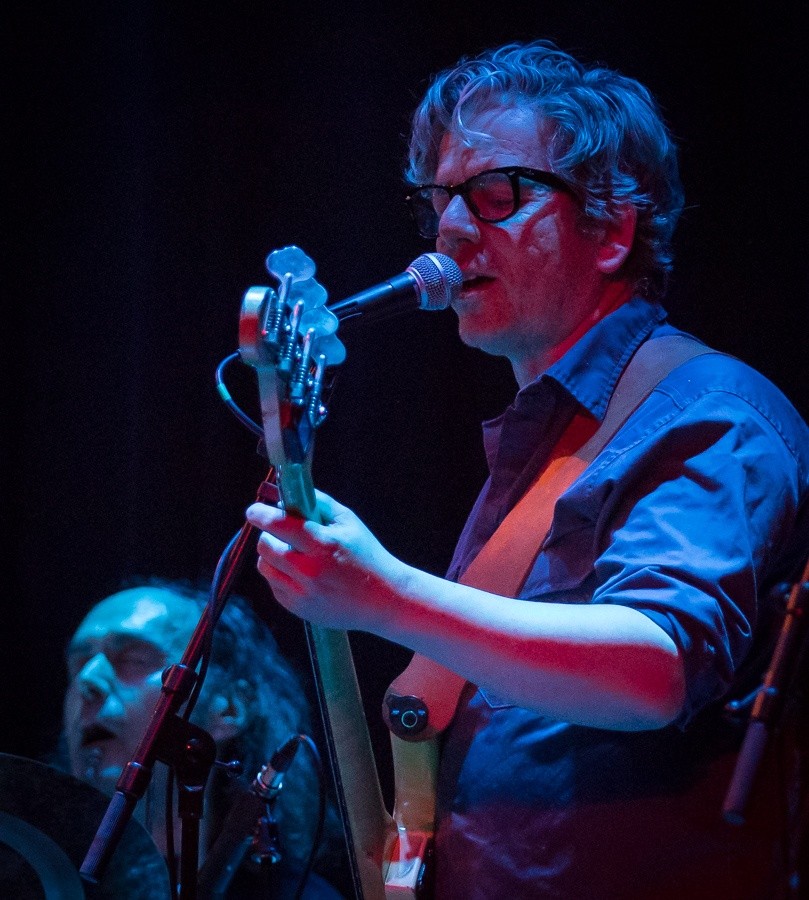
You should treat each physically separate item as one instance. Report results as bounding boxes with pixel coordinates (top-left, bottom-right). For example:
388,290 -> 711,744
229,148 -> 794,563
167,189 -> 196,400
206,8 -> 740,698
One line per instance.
246,39 -> 809,900
59,575 -> 346,900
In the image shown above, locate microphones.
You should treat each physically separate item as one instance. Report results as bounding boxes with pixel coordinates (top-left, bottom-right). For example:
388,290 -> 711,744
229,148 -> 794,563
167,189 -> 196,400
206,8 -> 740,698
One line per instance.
194,735 -> 301,900
238,254 -> 464,358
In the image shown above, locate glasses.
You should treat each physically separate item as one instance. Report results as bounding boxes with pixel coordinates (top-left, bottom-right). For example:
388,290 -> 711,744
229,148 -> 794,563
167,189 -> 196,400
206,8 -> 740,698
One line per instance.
404,166 -> 588,239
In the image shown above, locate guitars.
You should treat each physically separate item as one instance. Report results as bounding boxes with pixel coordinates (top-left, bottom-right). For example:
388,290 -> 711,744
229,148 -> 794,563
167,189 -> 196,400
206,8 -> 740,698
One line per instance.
235,244 -> 446,900
